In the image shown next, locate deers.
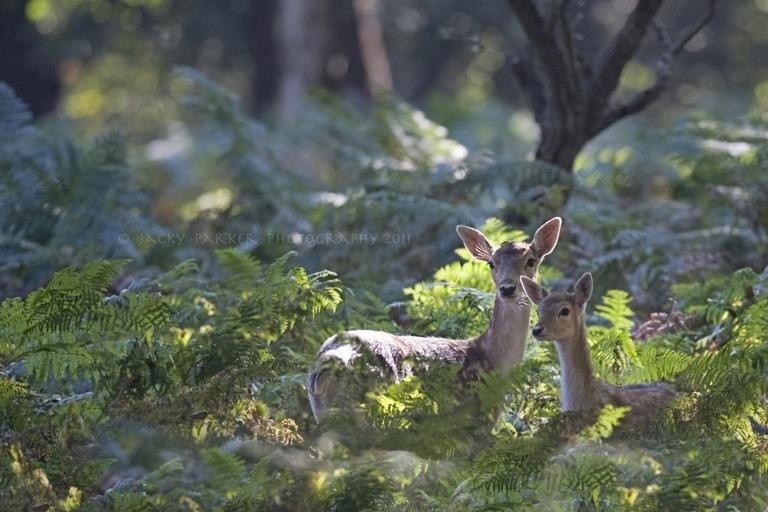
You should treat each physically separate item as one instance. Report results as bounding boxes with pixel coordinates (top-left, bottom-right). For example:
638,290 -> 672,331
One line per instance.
307,214 -> 563,438
520,271 -> 711,447
